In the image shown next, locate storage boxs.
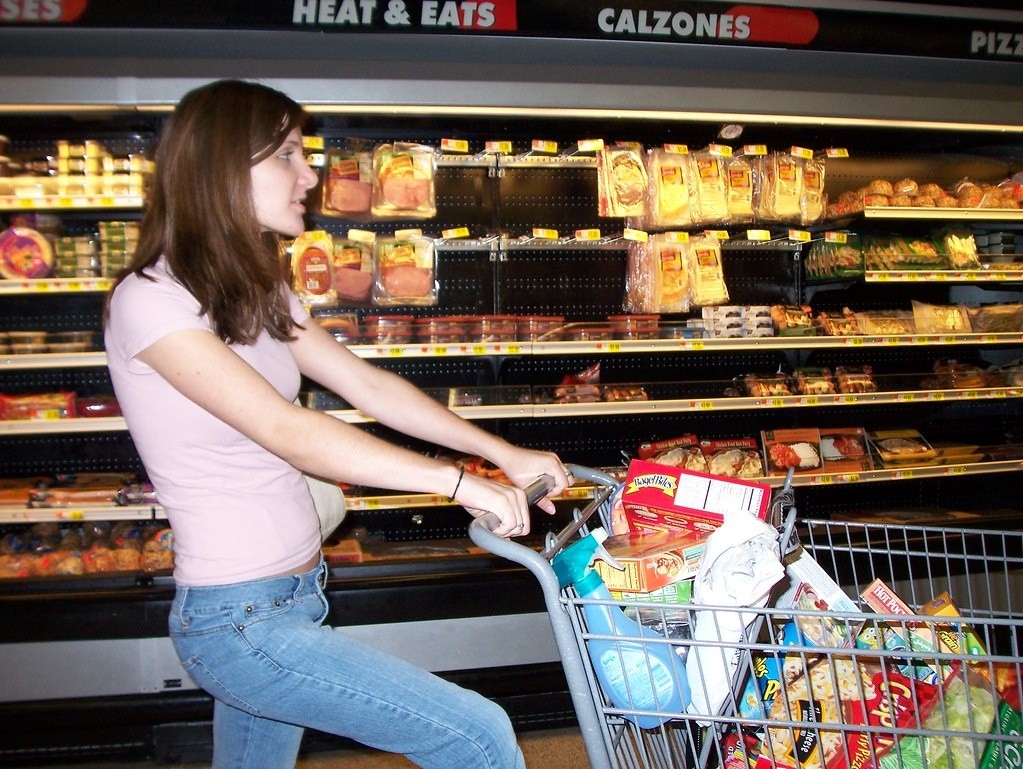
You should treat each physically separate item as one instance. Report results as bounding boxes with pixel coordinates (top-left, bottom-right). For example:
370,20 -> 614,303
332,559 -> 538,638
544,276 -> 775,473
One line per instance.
294,300 -> 777,347
971,229 -> 1019,266
3,130 -> 158,197
52,218 -> 140,278
2,328 -> 95,359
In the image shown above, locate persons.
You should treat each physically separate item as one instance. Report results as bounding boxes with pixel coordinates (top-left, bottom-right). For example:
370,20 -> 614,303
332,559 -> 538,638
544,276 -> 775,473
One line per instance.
103,79 -> 574,769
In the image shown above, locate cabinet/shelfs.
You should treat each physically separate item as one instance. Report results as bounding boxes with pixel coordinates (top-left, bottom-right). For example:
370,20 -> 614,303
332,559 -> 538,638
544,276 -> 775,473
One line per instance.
2,105 -> 1023,602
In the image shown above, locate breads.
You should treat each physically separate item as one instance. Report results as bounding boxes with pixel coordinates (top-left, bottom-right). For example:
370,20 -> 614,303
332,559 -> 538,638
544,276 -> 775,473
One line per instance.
824,179 -> 1023,220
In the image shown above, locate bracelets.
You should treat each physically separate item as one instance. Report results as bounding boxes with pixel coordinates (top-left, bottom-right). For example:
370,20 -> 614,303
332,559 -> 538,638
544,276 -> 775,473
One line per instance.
448,466 -> 464,503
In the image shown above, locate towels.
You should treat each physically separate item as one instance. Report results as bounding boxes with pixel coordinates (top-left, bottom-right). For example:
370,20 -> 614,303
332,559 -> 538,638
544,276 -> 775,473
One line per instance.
686,512 -> 785,727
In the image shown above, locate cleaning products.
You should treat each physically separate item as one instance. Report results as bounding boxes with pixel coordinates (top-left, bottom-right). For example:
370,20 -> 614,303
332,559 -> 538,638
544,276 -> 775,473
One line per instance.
551,527 -> 691,729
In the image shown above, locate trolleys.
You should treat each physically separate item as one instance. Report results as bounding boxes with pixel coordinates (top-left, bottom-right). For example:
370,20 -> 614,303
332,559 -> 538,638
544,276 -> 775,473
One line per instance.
469,464 -> 1022,769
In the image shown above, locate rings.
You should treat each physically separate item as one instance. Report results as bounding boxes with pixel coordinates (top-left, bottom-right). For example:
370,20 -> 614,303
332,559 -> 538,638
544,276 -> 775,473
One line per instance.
516,524 -> 524,528
565,471 -> 572,477
